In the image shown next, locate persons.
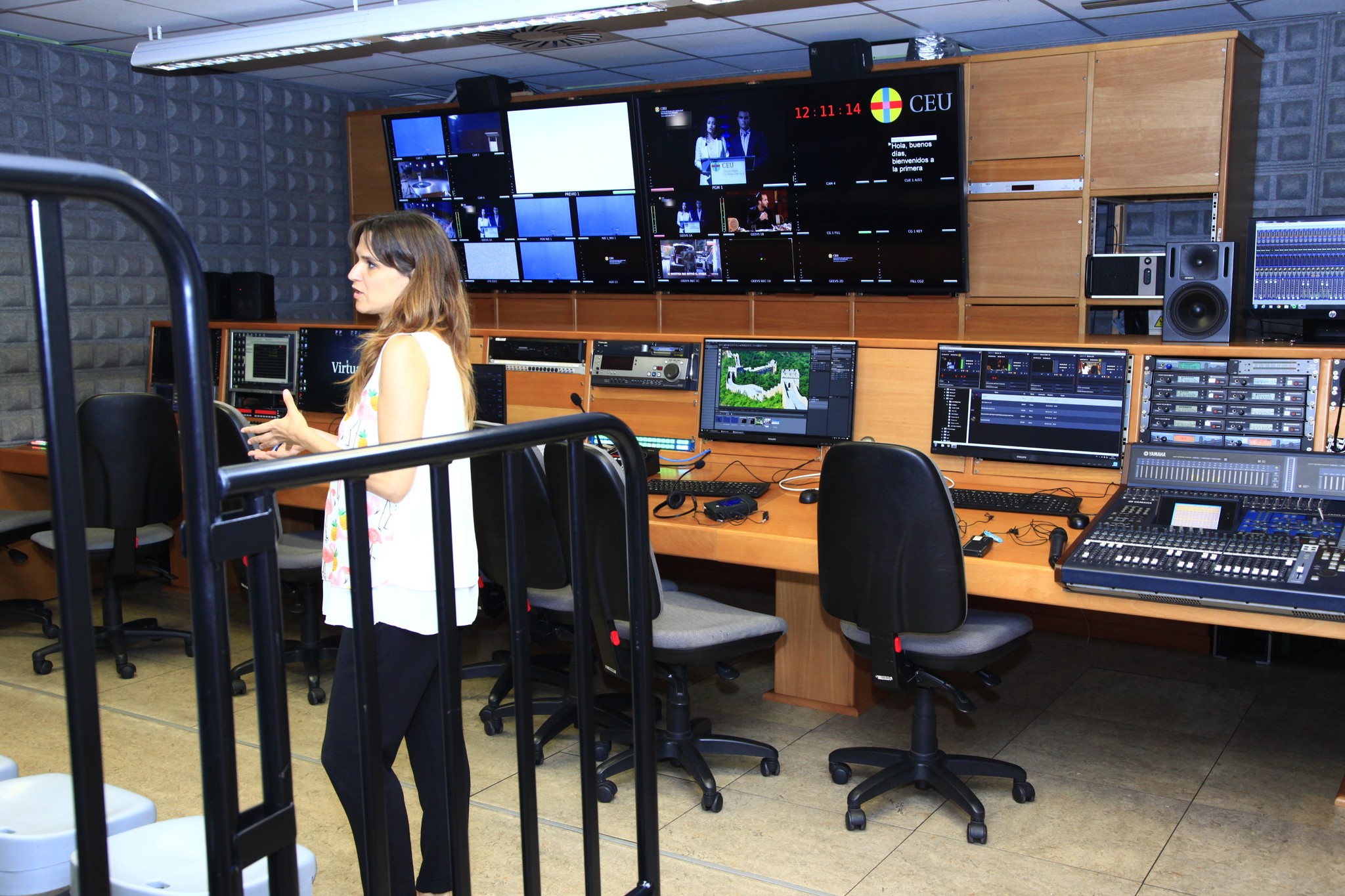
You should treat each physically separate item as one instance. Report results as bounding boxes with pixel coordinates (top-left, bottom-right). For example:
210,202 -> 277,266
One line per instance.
237,213 -> 482,896
676,199 -> 711,234
729,108 -> 769,170
694,115 -> 729,186
746,191 -> 777,230
680,246 -> 697,272
477,206 -> 506,238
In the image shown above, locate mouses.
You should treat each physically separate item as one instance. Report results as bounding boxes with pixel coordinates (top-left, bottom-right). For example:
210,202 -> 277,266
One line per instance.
800,489 -> 819,503
1068,512 -> 1089,529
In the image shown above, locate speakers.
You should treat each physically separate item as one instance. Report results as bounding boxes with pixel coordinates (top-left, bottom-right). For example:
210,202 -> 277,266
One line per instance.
1161,241 -> 1236,344
231,271 -> 275,322
201,271 -> 233,323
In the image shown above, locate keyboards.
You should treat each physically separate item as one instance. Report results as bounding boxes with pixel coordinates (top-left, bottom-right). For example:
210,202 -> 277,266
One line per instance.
647,479 -> 770,497
949,488 -> 1082,517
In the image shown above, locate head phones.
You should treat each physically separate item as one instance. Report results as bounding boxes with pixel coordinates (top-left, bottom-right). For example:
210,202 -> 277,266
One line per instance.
653,492 -> 698,518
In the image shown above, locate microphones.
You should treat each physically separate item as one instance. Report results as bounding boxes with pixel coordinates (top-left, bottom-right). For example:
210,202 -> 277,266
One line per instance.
1049,527 -> 1068,567
671,460 -> 705,492
570,392 -> 603,449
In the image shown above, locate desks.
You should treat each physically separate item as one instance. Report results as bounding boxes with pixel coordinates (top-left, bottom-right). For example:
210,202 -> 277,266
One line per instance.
0,434 -> 1345,725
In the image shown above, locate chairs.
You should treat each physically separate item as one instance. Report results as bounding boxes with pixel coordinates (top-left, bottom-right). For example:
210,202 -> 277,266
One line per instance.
541,442 -> 789,821
211,398 -> 342,707
467,414 -> 646,762
811,436 -> 1039,849
0,502 -> 60,643
27,390 -> 194,680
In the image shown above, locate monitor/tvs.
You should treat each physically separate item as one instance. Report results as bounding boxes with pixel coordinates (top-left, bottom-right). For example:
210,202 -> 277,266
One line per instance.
229,331 -> 297,396
931,342 -> 1129,468
297,328 -> 375,413
383,64 -> 970,296
697,338 -> 858,447
470,364 -> 507,423
